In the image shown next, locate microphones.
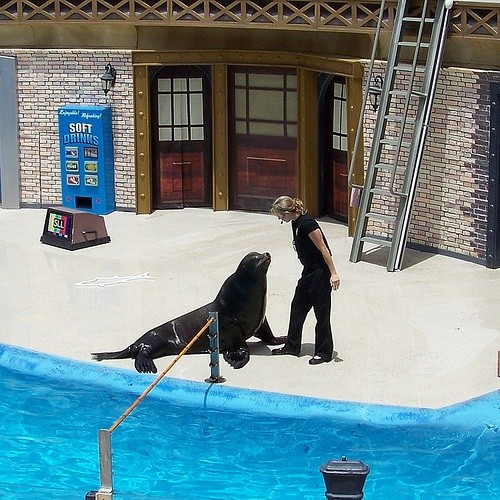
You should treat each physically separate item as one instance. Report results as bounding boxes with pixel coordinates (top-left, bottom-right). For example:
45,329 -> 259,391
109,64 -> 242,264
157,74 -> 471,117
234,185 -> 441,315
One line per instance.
280,213 -> 285,224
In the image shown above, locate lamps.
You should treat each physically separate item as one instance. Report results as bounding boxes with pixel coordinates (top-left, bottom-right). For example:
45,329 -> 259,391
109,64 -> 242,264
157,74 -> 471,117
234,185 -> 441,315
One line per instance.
100,64 -> 116,96
369,75 -> 383,113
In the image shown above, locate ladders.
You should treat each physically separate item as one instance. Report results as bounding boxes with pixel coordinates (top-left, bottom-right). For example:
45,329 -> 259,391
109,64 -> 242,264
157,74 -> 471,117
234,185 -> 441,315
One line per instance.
348,0 -> 454,271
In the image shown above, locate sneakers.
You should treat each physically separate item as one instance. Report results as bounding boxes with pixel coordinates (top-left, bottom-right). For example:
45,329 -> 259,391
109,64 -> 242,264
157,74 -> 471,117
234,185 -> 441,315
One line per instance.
271,347 -> 294,354
308,356 -> 324,365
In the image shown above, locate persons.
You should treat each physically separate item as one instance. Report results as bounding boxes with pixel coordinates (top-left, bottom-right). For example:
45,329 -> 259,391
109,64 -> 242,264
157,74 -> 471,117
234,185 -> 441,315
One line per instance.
268,194 -> 343,367
